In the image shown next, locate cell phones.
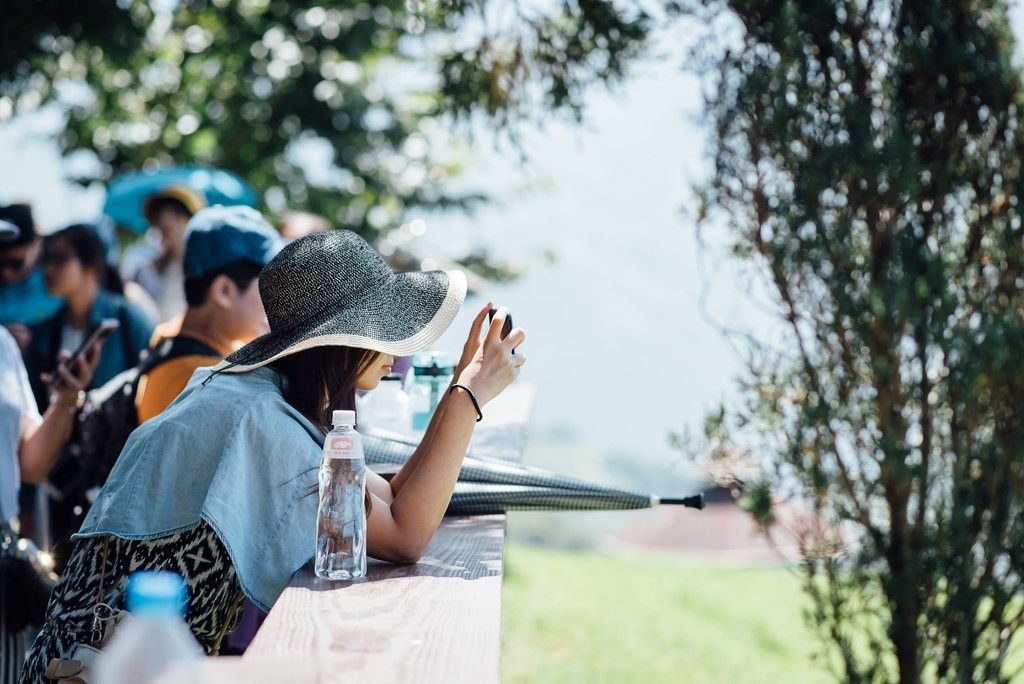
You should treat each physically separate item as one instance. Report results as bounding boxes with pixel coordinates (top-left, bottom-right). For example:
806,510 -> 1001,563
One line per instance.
489,310 -> 515,355
51,319 -> 119,386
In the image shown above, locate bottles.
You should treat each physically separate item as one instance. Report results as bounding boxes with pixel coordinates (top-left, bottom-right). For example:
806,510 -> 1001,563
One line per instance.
314,410 -> 368,580
89,572 -> 216,684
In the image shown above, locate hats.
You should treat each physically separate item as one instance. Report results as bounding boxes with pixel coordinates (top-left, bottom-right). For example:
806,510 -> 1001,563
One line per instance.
144,186 -> 206,219
183,204 -> 294,279
0,220 -> 20,244
210,229 -> 467,372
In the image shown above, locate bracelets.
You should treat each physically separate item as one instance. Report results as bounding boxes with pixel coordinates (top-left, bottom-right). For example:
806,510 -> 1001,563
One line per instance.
449,383 -> 483,423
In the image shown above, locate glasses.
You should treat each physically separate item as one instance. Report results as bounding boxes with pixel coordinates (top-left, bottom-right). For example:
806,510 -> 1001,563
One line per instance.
36,253 -> 79,266
0,248 -> 27,270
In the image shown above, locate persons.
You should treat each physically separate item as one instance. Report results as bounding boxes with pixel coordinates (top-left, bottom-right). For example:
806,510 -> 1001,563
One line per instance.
1,185 -> 295,683
16,227 -> 527,684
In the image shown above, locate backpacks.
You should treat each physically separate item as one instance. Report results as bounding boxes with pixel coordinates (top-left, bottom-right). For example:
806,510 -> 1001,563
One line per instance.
60,336 -> 227,509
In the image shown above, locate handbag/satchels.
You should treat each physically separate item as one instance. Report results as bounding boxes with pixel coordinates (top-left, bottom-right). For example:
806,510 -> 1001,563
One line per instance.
0,520 -> 61,637
45,644 -> 107,684
